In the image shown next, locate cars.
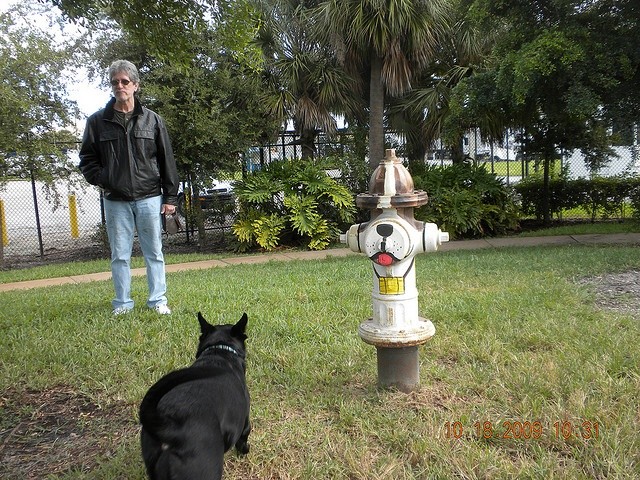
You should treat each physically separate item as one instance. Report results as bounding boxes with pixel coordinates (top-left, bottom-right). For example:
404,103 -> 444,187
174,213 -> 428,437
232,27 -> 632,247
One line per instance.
178,177 -> 238,217
0,152 -> 72,180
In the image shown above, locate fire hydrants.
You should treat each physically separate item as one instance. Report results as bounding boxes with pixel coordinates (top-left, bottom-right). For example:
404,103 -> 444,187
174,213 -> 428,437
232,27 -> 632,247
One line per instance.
338,147 -> 451,393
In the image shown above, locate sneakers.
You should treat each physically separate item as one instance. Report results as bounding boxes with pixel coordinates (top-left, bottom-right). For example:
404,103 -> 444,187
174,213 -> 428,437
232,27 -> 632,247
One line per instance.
149,303 -> 172,317
111,306 -> 131,315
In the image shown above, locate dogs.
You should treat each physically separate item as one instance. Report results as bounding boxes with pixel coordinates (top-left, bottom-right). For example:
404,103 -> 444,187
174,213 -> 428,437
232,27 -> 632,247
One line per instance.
139,311 -> 252,480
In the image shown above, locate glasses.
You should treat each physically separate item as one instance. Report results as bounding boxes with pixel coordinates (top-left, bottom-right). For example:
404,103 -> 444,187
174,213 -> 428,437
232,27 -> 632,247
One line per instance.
111,79 -> 132,86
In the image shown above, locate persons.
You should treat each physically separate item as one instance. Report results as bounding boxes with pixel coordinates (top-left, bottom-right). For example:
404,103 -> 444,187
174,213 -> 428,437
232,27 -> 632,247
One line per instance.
77,59 -> 180,318
